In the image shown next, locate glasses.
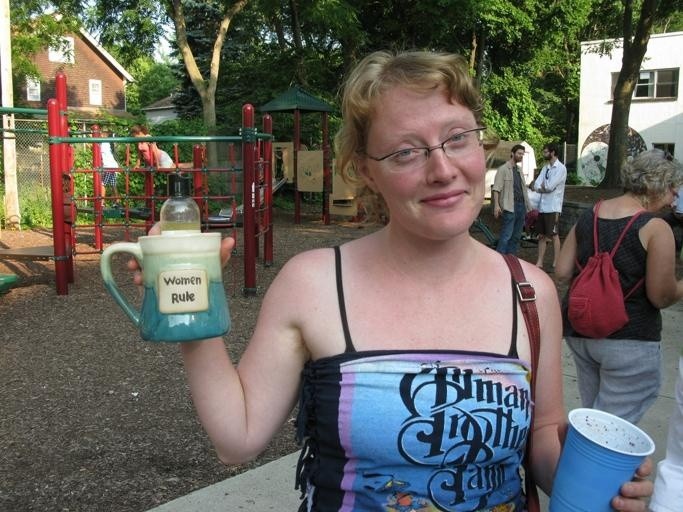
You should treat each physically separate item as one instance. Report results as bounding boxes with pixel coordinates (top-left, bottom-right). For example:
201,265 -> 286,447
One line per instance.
357,126 -> 487,173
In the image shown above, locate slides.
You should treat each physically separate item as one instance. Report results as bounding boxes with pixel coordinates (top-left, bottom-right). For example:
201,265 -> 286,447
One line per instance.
208,178 -> 288,222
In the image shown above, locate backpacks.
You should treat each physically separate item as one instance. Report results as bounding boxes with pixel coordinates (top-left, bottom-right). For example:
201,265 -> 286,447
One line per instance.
568,199 -> 647,338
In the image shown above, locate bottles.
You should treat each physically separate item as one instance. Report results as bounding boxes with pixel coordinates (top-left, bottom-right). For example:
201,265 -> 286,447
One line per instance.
160,169 -> 203,236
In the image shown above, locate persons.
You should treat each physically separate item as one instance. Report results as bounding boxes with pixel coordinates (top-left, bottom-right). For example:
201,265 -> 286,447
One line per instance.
661,173 -> 683,252
553,151 -> 683,424
99,130 -> 121,207
491,145 -> 534,254
130,122 -> 195,173
533,143 -> 567,269
124,48 -> 657,511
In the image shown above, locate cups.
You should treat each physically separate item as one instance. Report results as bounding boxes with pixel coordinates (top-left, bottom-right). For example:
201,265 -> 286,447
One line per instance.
548,406 -> 658,509
98,232 -> 231,344
